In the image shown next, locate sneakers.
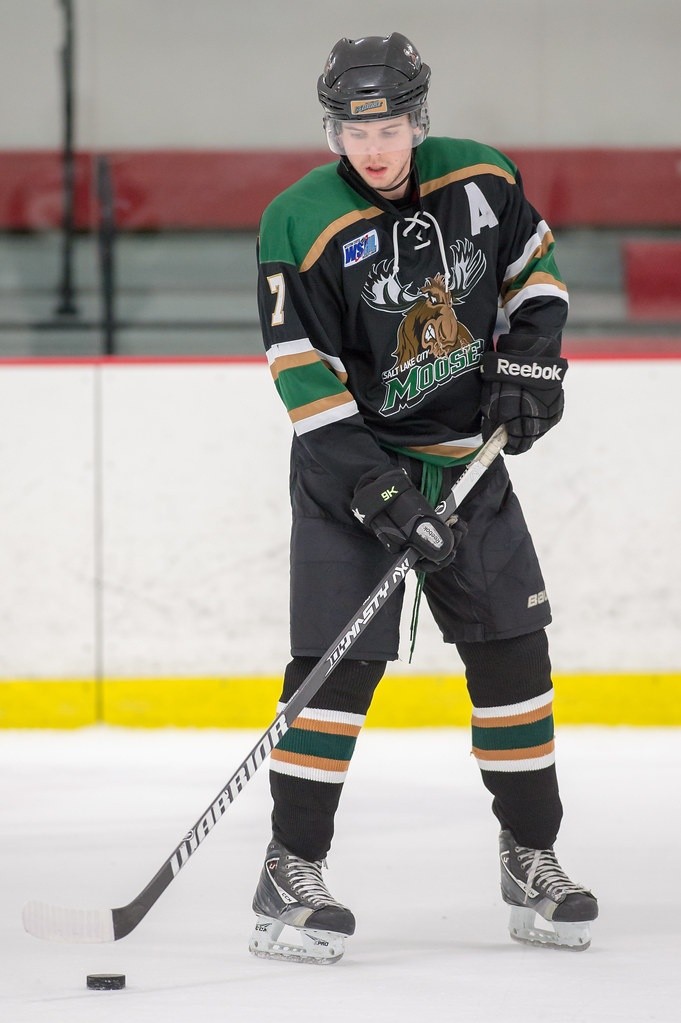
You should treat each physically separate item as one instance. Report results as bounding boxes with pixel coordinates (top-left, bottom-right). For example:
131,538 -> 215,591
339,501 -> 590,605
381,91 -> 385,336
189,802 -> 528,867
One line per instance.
496,826 -> 600,946
249,839 -> 356,964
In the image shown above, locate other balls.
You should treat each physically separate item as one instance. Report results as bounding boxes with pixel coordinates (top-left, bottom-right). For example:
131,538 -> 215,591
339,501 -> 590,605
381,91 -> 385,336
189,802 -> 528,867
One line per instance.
86,973 -> 126,991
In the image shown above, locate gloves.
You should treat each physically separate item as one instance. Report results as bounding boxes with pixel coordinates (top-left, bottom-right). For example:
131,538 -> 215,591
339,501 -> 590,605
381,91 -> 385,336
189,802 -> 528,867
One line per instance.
350,467 -> 470,572
481,331 -> 569,456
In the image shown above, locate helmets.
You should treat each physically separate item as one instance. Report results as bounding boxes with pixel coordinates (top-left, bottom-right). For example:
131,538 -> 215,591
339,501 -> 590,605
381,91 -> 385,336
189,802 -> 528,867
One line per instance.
317,31 -> 431,124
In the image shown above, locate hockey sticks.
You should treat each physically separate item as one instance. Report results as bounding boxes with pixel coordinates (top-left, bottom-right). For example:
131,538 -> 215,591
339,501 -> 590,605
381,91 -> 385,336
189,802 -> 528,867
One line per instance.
109,423 -> 510,942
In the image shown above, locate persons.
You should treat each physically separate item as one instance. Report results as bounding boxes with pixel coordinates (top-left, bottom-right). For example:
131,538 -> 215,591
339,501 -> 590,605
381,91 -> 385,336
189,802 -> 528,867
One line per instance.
252,33 -> 602,963
